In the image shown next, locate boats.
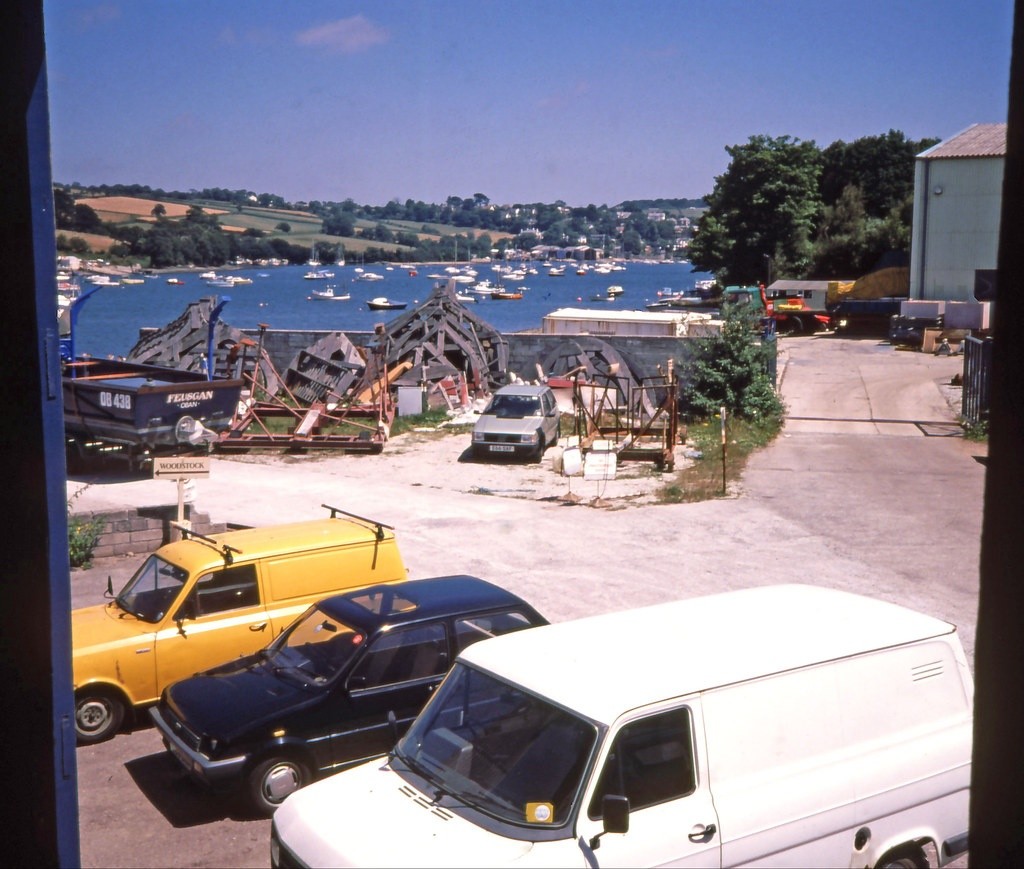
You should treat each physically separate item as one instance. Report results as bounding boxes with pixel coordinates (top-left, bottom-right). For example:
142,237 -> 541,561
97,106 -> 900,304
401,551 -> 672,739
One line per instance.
366,297 -> 409,311
59,283 -> 246,447
312,284 -> 352,300
55,235 -> 726,336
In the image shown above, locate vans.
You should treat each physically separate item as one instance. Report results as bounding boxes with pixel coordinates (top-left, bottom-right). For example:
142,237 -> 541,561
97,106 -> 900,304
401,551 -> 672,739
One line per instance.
269,582 -> 973,869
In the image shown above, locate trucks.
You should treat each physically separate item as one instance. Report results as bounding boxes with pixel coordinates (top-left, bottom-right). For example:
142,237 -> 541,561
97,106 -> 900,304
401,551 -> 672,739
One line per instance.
718,281 -> 831,336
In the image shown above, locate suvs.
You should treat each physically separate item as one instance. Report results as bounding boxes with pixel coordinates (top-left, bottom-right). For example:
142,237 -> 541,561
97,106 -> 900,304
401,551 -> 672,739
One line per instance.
470,384 -> 561,463
149,569 -> 551,819
71,503 -> 416,743
